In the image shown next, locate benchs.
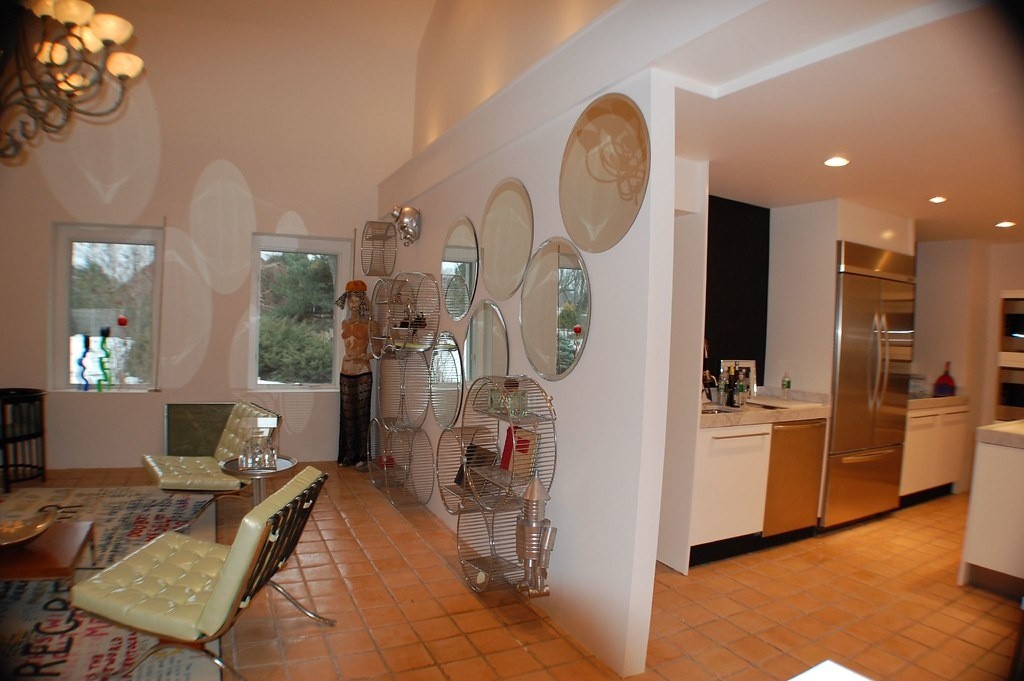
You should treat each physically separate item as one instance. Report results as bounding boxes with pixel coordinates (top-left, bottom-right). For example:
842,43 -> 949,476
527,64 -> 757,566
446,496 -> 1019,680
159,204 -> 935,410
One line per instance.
143,400 -> 284,532
68,466 -> 334,681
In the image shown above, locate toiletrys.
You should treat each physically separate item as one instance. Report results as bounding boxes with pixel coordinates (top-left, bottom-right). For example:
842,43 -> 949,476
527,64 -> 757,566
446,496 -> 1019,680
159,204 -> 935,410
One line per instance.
718,361 -> 729,406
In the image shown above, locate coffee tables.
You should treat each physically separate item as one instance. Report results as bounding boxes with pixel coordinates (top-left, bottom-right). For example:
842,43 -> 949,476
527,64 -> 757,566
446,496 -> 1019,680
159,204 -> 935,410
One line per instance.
0,521 -> 95,608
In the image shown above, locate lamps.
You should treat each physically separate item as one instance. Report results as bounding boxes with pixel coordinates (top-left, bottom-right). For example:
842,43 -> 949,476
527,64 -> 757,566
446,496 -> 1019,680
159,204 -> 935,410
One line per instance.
0,0 -> 145,162
576,101 -> 645,203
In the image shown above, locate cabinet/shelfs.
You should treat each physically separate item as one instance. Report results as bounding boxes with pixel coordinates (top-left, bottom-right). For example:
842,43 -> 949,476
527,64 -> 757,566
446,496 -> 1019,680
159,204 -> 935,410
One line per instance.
690,419 -> 772,546
900,407 -> 969,500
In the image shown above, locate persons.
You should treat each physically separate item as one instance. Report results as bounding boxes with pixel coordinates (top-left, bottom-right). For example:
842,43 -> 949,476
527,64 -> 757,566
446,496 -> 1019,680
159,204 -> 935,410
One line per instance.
337,281 -> 383,469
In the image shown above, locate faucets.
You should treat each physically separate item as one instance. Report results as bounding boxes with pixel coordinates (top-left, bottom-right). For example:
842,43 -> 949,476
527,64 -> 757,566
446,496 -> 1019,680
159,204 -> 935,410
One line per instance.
701,337 -> 708,404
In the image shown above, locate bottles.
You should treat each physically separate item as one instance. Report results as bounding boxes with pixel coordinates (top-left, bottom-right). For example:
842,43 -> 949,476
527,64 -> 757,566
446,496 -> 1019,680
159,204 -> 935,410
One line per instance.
729,361 -> 746,408
932,361 -> 956,398
718,366 -> 729,405
781,371 -> 791,400
113,315 -> 132,392
737,375 -> 746,409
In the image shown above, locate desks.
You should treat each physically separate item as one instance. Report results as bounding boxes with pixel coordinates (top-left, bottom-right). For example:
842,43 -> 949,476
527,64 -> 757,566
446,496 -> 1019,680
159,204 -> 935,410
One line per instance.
0,388 -> 47,494
220,454 -> 298,507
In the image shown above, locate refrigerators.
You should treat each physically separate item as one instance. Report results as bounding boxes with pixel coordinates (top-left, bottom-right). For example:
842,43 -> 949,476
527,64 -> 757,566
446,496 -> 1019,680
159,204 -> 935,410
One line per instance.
763,199 -> 916,527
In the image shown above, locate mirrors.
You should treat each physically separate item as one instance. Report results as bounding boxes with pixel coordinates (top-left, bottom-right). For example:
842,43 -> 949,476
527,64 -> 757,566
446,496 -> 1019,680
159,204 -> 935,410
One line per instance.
519,237 -> 591,382
462,298 -> 509,415
478,177 -> 534,301
431,331 -> 464,430
440,216 -> 478,322
559,92 -> 651,254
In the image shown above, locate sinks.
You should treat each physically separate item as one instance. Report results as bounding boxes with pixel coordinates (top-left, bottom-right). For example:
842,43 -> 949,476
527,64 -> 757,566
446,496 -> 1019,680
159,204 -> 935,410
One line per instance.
701,405 -> 742,415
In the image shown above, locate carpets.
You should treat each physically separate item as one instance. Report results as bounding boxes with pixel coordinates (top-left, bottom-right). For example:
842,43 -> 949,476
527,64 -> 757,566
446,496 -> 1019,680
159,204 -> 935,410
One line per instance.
0,487 -> 223,681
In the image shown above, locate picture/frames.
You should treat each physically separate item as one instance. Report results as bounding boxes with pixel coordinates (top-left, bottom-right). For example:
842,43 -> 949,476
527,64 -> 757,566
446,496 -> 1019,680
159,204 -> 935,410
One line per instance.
721,360 -> 757,398
